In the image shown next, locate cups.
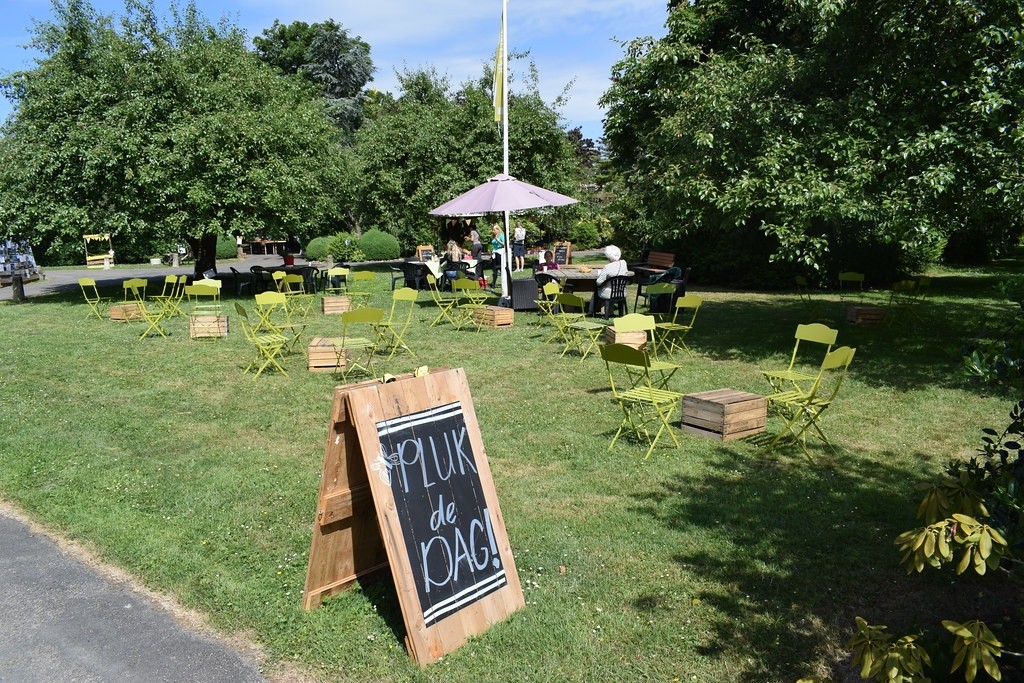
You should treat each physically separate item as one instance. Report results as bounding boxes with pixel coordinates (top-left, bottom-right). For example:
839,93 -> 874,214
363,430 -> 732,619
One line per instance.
543,266 -> 548,272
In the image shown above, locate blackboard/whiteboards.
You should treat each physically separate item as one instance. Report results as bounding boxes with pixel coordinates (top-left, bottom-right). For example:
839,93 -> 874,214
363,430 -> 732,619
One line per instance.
552,242 -> 572,265
303,367 -> 526,666
415,245 -> 433,261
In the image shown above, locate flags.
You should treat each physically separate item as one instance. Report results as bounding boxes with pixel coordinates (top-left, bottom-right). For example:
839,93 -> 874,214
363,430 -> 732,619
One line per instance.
492,1 -> 509,122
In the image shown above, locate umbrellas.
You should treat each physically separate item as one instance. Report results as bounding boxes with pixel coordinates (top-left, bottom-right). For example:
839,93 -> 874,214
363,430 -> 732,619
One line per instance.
427,172 -> 580,309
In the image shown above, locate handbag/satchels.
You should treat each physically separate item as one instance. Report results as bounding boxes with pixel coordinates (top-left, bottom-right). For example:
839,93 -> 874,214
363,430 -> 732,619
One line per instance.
516,227 -> 526,242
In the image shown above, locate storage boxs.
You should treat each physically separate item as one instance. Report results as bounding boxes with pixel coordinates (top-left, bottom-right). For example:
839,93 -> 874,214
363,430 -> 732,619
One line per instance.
190,315 -> 231,338
476,304 -> 514,327
605,326 -> 647,350
322,297 -> 352,314
308,338 -> 345,372
847,305 -> 884,327
109,306 -> 143,320
681,388 -> 768,442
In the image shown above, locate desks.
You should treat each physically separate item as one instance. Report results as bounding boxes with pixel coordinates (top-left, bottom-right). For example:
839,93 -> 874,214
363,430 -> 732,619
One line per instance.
265,265 -> 308,277
536,268 -> 600,293
410,259 -> 477,288
249,240 -> 286,255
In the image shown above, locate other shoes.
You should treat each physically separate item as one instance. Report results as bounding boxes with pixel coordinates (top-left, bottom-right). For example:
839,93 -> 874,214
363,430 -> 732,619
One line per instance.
582,314 -> 593,318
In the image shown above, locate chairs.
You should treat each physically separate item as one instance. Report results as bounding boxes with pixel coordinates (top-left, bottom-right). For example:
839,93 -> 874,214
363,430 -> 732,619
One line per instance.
885,276 -> 931,328
767,345 -> 856,471
839,272 -> 864,305
613,312 -> 683,413
796,276 -> 836,327
77,262 -> 490,383
599,343 -> 685,460
764,323 -> 838,423
509,266 -> 704,360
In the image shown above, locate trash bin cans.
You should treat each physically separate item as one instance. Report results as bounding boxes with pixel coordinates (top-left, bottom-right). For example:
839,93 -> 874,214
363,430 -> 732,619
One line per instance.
649,267 -> 683,313
173,254 -> 180,267
104,258 -> 110,270
513,279 -> 541,311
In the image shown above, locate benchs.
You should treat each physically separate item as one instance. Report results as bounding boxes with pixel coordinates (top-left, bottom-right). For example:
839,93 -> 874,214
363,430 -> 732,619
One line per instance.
634,251 -> 674,275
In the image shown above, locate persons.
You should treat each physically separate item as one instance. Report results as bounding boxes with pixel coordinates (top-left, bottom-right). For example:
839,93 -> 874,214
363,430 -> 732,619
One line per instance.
538,251 -> 559,270
487,225 -> 513,289
437,240 -> 462,292
462,231 -> 485,289
585,244 -> 628,318
512,221 -> 526,272
